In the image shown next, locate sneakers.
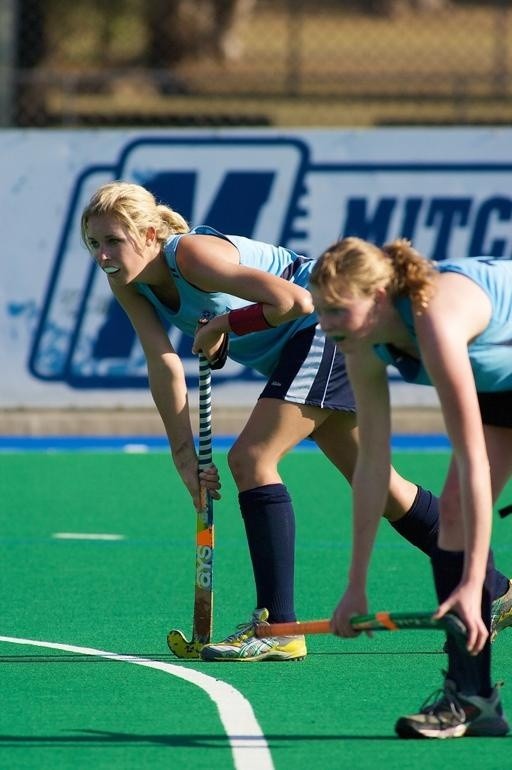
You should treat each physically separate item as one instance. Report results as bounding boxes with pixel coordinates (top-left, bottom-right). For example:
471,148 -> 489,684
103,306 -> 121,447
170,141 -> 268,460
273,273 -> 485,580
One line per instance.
443,579 -> 512,653
201,607 -> 307,662
396,678 -> 508,739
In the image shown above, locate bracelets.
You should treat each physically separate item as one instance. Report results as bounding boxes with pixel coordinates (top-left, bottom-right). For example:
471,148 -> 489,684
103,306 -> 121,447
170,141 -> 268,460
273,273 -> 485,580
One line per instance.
228,303 -> 277,336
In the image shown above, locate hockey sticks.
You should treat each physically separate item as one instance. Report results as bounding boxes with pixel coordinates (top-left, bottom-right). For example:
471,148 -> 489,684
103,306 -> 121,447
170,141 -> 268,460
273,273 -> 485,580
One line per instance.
253,612 -> 469,653
167,317 -> 215,657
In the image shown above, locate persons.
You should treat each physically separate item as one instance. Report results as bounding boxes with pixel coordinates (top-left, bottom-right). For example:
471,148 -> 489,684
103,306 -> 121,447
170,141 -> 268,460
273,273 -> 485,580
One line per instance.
82,182 -> 512,663
309,237 -> 512,739
